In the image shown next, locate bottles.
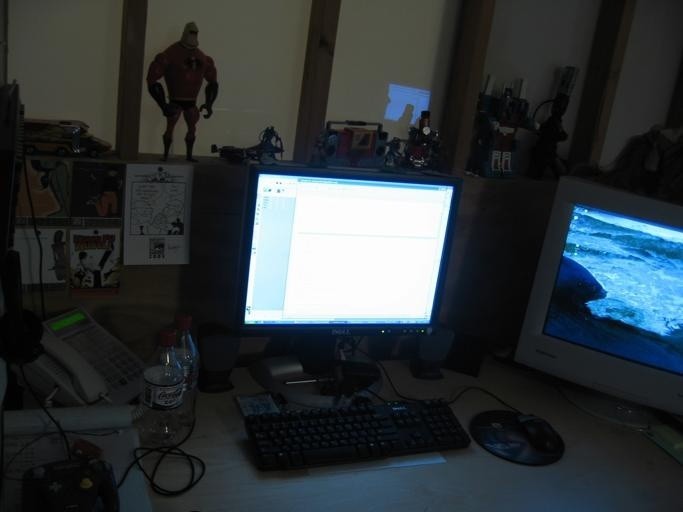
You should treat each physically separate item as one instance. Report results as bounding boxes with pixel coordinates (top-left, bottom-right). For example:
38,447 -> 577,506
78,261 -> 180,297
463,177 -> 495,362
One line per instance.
415,109 -> 432,148
478,121 -> 517,177
140,308 -> 199,449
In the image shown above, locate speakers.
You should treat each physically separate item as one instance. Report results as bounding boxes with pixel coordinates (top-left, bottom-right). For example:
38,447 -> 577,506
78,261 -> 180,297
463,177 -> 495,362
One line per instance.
196,321 -> 240,394
411,321 -> 456,379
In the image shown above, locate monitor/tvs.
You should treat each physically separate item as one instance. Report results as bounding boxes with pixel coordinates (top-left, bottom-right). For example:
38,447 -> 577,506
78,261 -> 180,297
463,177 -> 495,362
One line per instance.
513,173 -> 683,431
231,159 -> 464,408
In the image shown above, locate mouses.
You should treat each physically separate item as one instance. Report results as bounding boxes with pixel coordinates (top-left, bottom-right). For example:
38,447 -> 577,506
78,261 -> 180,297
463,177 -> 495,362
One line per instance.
514,412 -> 558,453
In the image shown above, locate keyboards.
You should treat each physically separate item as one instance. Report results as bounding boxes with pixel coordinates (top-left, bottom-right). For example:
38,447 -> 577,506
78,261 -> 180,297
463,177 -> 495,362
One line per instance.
244,397 -> 471,471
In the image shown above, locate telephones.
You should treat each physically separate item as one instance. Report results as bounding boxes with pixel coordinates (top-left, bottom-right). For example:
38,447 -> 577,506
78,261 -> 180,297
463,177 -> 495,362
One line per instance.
22,306 -> 149,408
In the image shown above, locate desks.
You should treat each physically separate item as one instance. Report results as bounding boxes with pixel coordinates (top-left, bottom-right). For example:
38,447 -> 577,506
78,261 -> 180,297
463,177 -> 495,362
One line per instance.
139,357 -> 683,512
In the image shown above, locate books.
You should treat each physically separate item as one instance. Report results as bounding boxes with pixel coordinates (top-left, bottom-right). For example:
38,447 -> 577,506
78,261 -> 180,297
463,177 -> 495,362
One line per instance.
0,404 -> 153,512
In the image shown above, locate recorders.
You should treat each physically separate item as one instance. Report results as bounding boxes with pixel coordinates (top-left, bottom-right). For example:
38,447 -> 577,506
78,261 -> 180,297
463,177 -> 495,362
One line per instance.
325,118 -> 389,169
